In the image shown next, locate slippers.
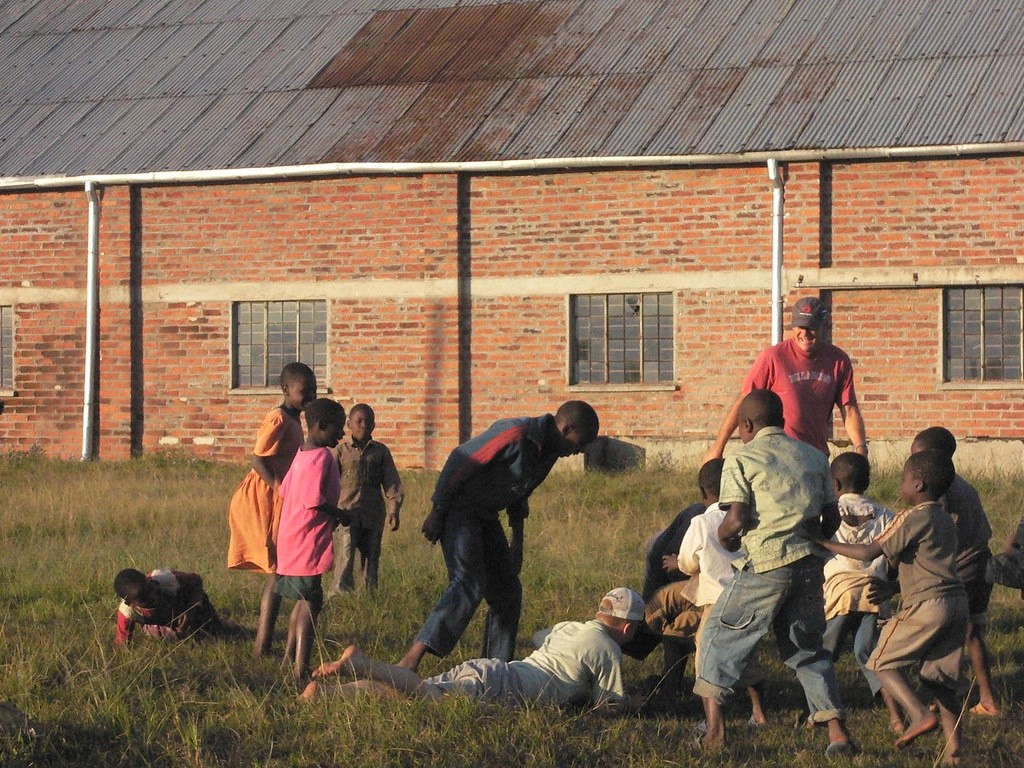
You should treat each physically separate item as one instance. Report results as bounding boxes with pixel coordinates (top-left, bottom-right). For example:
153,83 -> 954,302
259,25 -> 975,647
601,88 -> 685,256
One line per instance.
746,716 -> 769,727
698,719 -> 707,729
969,703 -> 1008,718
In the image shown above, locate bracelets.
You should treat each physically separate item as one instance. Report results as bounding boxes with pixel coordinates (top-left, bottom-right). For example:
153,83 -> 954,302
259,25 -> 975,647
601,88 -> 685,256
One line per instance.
855,442 -> 868,453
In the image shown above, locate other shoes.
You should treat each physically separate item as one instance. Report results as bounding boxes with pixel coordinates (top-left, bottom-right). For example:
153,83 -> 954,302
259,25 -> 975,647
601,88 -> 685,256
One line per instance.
825,740 -> 857,759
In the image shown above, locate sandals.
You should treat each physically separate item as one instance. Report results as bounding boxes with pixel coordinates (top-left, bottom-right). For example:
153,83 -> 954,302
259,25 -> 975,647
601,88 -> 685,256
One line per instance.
887,721 -> 905,733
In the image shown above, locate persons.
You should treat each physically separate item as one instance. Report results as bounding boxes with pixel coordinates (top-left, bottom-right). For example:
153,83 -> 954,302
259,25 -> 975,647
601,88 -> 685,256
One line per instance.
701,298 -> 869,462
275,398 -> 351,679
295,588 -> 646,712
628,389 -> 1024,768
329,404 -> 404,599
394,401 -> 598,671
113,568 -> 220,645
227,362 -> 319,653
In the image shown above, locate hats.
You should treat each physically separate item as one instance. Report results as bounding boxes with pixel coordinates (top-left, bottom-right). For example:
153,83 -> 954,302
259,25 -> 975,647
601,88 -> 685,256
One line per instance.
791,298 -> 828,330
596,587 -> 647,621
